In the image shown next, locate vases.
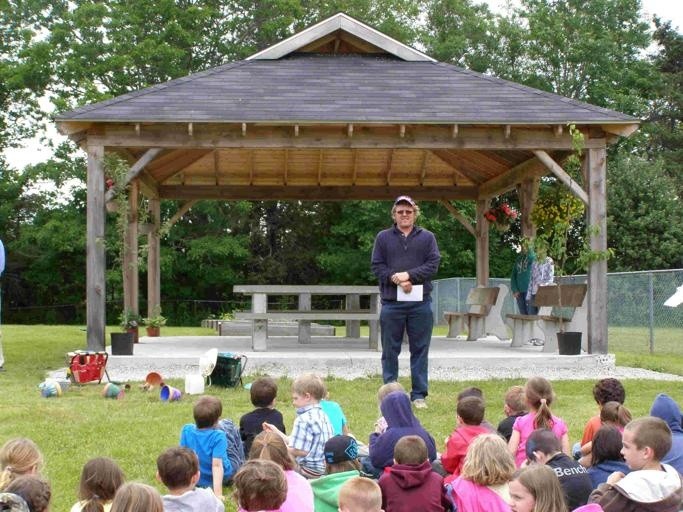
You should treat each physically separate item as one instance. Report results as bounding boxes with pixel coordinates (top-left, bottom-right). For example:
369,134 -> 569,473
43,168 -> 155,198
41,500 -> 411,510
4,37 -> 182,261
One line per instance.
496,223 -> 511,233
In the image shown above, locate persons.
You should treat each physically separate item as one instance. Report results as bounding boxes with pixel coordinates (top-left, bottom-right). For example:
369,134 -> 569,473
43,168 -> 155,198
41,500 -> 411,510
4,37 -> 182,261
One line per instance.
509,464 -> 604,512
377,435 -> 454,512
442,396 -> 491,474
375,383 -> 410,435
337,474 -> 385,512
587,425 -> 629,491
156,447 -> 225,512
371,197 -> 440,409
262,375 -> 334,478
520,428 -> 592,508
587,417 -> 683,512
512,238 -> 535,315
445,433 -> 514,512
0,473 -> 51,512
69,457 -> 122,512
580,401 -> 632,467
508,376 -> 569,464
317,381 -> 349,438
180,395 -> 242,501
249,429 -> 314,512
233,459 -> 288,512
110,481 -> 163,512
456,386 -> 507,444
0,236 -> 6,373
582,378 -> 631,447
527,239 -> 554,347
497,386 -> 529,441
0,437 -> 44,493
359,392 -> 436,474
240,377 -> 285,457
650,393 -> 683,477
309,435 -> 362,512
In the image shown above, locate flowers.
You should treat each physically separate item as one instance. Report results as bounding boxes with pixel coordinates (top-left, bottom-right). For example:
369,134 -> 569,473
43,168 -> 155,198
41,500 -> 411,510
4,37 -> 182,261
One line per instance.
482,193 -> 517,224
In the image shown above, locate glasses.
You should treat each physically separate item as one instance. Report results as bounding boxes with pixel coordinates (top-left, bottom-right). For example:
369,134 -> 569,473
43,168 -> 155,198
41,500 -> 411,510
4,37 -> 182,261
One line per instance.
397,210 -> 414,215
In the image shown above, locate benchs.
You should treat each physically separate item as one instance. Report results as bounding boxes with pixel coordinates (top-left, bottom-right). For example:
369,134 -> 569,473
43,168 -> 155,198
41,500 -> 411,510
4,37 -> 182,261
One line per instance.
232,285 -> 383,313
232,310 -> 379,350
445,287 -> 500,341
505,284 -> 587,348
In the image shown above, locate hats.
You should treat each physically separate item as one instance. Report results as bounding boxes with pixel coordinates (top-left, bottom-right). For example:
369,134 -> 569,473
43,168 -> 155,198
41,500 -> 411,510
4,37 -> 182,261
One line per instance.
324,434 -> 358,462
394,195 -> 415,207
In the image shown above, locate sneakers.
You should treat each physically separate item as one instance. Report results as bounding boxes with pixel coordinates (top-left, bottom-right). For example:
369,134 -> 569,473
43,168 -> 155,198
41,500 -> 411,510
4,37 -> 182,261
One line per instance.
414,396 -> 427,410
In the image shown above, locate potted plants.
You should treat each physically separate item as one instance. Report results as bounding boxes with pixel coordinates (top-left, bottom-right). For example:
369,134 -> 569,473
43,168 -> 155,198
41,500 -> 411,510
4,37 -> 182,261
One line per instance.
516,120 -> 616,356
121,311 -> 142,343
143,305 -> 167,338
